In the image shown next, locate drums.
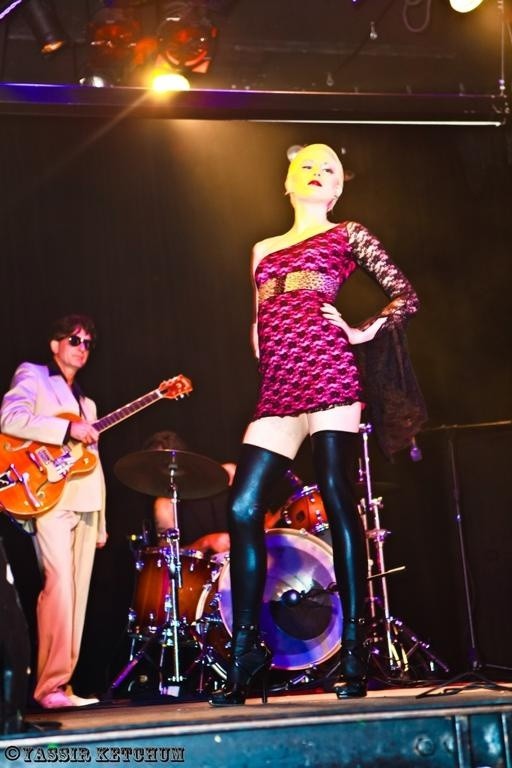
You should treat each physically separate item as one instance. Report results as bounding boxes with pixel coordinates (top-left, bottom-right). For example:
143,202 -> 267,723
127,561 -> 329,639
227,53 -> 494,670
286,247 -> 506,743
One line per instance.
283,485 -> 332,534
131,552 -> 224,632
196,528 -> 341,669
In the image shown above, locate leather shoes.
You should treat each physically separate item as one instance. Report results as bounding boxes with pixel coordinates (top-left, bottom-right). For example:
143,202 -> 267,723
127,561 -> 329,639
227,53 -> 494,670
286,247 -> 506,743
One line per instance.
41,691 -> 101,709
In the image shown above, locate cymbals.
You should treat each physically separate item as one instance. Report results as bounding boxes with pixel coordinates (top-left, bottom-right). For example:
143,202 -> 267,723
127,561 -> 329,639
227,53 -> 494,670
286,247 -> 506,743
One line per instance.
115,446 -> 228,499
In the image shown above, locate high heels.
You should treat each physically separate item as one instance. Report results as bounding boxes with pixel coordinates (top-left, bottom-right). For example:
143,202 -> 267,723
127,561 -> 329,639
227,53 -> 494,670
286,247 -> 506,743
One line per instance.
209,637 -> 272,707
335,644 -> 371,699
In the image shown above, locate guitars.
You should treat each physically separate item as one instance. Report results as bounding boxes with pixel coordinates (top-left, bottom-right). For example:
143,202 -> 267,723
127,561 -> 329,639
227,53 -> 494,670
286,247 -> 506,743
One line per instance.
0,373 -> 194,515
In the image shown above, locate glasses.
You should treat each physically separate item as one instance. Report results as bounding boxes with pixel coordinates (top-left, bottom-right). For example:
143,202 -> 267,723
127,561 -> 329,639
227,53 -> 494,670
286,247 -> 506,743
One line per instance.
67,333 -> 95,350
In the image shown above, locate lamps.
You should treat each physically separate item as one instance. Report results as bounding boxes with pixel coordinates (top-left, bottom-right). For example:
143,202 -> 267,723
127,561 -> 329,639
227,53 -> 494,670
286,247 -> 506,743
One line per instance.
20,0 -> 218,91
449,0 -> 483,13
370,21 -> 378,40
0,0 -> 510,128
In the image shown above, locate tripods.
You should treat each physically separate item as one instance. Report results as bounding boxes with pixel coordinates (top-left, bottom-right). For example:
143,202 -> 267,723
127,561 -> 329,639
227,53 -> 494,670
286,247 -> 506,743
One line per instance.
414,435 -> 512,699
323,505 -> 450,679
103,536 -> 229,700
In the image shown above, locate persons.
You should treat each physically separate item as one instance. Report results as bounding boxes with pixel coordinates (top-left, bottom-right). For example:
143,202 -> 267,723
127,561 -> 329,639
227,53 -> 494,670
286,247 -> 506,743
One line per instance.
207,140 -> 420,705
144,428 -> 238,557
1,313 -> 112,708
